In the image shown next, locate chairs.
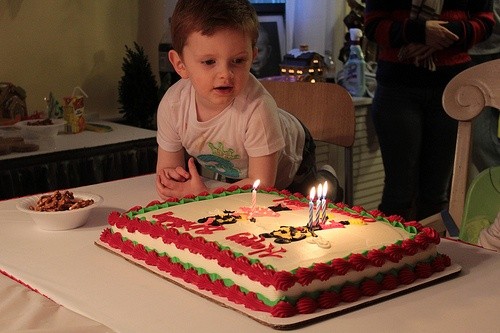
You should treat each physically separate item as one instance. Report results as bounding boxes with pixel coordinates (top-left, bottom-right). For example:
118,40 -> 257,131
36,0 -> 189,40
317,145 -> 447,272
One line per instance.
440,59 -> 500,240
259,78 -> 357,209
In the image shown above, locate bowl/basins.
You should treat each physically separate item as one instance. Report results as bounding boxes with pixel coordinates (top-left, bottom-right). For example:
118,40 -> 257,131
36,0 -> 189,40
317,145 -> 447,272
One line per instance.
16,192 -> 104,231
13,118 -> 68,146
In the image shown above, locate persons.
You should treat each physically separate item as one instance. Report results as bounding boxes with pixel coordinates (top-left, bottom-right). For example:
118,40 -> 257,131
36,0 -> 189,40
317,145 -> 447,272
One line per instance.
156,0 -> 344,204
250,23 -> 280,79
362,0 -> 500,222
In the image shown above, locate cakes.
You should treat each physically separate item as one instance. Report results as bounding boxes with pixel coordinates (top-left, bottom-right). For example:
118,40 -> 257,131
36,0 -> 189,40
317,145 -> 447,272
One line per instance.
99,184 -> 452,316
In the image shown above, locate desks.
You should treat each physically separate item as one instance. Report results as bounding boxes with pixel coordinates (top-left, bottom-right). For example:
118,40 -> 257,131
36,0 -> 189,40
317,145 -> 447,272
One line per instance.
313,96 -> 389,213
0,116 -> 160,201
0,170 -> 500,333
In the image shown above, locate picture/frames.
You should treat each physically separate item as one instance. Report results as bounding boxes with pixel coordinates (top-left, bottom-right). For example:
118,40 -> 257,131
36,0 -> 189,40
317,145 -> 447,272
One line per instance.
246,2 -> 289,81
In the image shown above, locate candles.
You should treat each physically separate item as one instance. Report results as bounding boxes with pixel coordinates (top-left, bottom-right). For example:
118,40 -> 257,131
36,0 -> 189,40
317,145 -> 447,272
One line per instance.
316,183 -> 323,226
322,180 -> 329,224
308,184 -> 316,228
249,178 -> 261,220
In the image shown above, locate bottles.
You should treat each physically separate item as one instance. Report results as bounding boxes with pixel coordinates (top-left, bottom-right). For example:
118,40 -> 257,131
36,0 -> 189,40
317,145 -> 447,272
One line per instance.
323,49 -> 337,85
158,17 -> 181,92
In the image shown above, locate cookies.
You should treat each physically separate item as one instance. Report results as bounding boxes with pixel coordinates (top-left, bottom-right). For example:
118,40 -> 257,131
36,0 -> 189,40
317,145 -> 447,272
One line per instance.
29,190 -> 95,210
